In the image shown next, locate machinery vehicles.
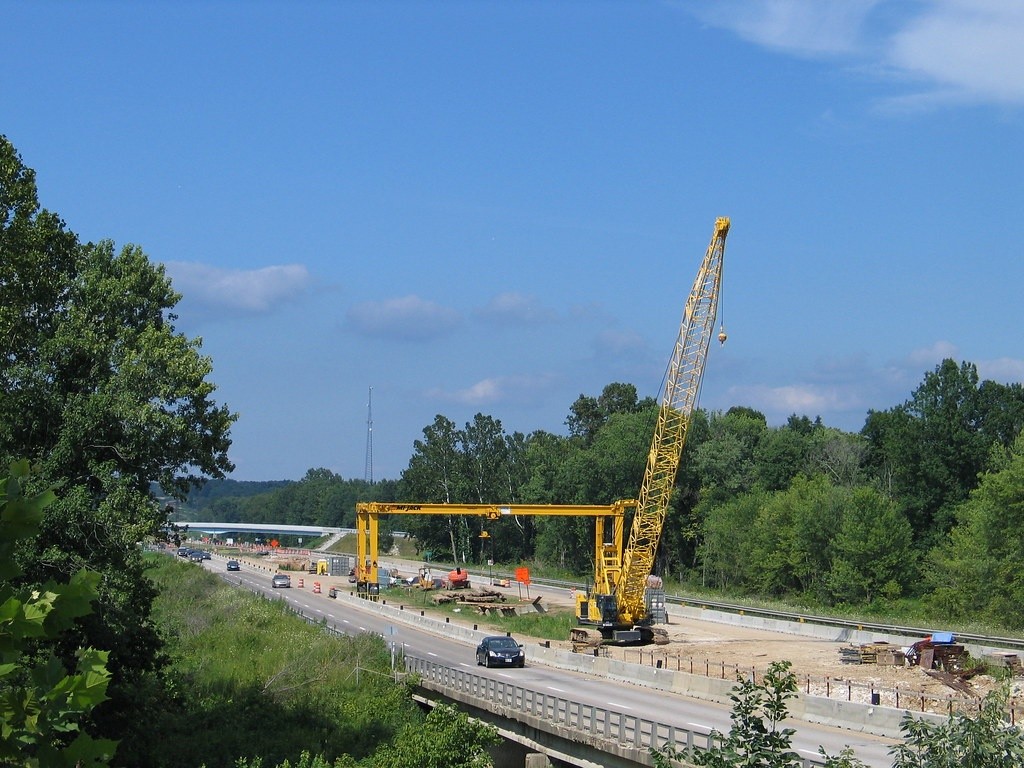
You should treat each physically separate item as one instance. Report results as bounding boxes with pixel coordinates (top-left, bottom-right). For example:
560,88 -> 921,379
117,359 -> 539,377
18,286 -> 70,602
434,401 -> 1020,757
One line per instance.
409,568 -> 435,592
439,569 -> 471,591
570,213 -> 733,644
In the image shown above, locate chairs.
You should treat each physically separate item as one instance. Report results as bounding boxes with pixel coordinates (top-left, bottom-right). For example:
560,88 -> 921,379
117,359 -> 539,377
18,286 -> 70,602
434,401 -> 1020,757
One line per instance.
491,642 -> 499,647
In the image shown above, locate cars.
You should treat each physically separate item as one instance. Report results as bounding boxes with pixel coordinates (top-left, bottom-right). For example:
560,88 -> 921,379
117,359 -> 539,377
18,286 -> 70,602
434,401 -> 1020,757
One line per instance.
177,547 -> 212,564
157,543 -> 166,549
227,560 -> 240,571
475,636 -> 526,668
272,574 -> 291,588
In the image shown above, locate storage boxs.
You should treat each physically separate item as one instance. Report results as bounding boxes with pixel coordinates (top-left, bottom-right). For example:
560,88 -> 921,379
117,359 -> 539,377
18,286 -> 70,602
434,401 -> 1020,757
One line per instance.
326,557 -> 349,575
